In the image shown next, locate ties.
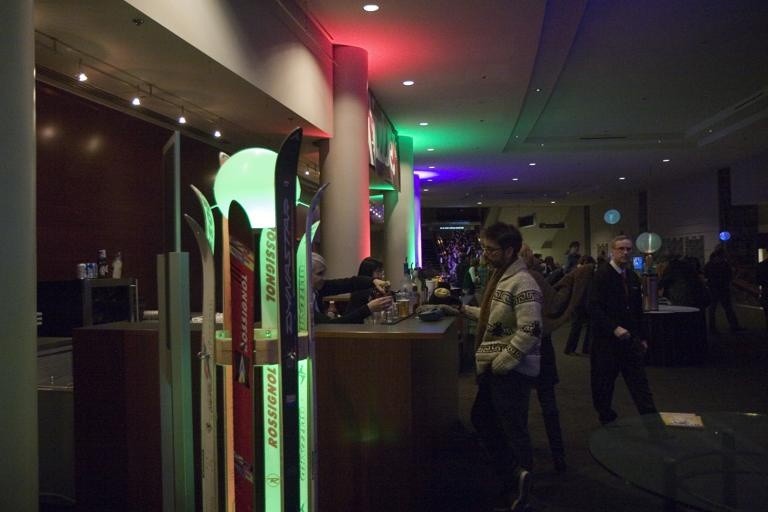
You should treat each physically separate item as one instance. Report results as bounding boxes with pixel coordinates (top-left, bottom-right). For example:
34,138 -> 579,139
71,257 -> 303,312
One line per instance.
620,270 -> 629,310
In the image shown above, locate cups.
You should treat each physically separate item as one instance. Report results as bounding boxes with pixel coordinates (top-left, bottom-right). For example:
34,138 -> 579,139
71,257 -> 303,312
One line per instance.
395,291 -> 411,317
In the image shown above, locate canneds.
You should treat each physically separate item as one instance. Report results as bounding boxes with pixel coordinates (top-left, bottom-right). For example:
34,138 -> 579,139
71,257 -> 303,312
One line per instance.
77,263 -> 98,279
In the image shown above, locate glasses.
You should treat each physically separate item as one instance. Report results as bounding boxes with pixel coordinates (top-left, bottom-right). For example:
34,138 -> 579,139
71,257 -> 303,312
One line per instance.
613,247 -> 632,254
480,244 -> 502,253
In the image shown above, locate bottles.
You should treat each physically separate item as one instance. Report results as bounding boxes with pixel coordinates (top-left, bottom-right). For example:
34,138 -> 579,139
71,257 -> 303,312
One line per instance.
327,300 -> 337,318
96,249 -> 109,279
400,262 -> 426,315
109,250 -> 123,280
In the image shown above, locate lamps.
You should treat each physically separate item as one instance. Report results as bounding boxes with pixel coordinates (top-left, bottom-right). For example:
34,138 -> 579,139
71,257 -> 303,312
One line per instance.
214,147 -> 302,231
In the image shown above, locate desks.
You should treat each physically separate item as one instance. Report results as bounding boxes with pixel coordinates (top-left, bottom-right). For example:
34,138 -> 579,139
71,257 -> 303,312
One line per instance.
35,277 -> 136,339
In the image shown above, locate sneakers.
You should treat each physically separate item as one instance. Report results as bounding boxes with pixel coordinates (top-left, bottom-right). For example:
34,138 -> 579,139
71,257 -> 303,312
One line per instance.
508,468 -> 537,511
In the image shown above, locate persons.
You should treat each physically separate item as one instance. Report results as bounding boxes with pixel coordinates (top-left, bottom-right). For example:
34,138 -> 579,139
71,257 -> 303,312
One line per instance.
345,255 -> 401,320
564,256 -> 596,356
404,265 -> 428,319
659,257 -> 710,331
517,244 -> 592,476
704,245 -> 745,334
534,253 -> 553,277
311,251 -> 394,323
464,258 -> 481,294
477,256 -> 489,282
452,221 -> 544,509
585,235 -> 663,428
565,242 -> 581,273
